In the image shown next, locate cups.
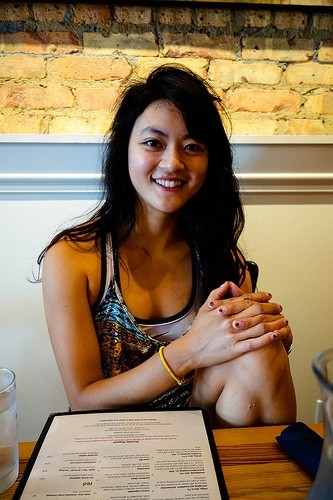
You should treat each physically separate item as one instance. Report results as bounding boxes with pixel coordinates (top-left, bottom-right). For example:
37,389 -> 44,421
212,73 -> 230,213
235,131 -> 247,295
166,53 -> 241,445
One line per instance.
0,366 -> 20,494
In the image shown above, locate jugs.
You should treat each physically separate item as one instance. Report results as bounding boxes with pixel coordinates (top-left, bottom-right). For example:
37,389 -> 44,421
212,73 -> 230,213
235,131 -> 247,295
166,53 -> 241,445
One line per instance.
303,347 -> 333,500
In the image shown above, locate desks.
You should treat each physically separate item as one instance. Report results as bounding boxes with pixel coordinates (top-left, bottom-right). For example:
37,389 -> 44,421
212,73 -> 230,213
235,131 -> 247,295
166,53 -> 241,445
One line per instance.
2,417 -> 326,499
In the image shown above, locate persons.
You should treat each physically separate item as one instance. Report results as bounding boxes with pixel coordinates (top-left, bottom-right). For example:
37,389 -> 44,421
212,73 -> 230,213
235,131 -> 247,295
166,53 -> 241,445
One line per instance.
24,62 -> 297,427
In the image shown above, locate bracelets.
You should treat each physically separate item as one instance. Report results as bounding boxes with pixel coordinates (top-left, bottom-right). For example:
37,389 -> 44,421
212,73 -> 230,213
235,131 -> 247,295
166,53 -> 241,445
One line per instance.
159,347 -> 185,386
